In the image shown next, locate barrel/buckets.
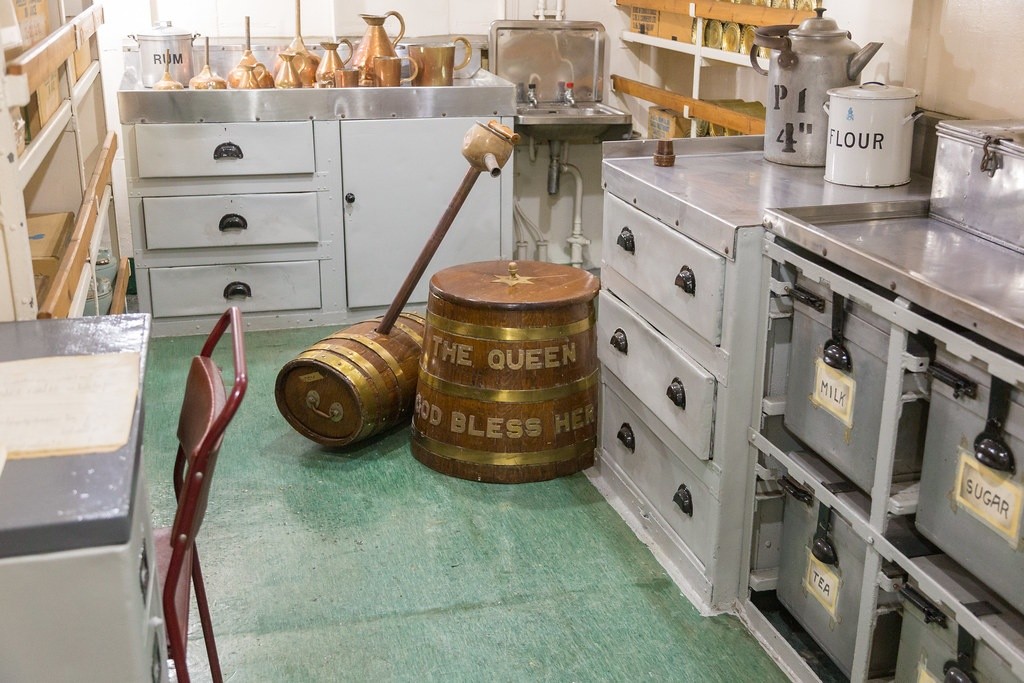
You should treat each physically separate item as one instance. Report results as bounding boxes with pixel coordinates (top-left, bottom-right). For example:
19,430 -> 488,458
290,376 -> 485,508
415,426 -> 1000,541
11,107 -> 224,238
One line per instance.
275,311 -> 423,449
414,263 -> 596,483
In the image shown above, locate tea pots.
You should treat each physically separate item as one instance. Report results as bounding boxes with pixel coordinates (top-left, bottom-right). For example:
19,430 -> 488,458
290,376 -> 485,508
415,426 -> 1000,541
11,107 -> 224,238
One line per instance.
750,7 -> 881,168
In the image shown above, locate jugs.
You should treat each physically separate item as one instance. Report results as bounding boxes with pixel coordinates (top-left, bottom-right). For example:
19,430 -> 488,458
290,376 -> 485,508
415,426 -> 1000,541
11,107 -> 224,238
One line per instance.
351,12 -> 405,82
236,63 -> 266,89
274,52 -> 306,88
315,39 -> 352,82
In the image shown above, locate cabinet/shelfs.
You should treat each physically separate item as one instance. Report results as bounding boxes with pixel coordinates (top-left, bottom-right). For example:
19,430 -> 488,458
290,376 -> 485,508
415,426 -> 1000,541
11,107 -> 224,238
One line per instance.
581,106 -> 1024,683
0,311 -> 168,683
609,0 -> 818,141
0,0 -> 132,323
115,118 -> 331,339
334,113 -> 524,325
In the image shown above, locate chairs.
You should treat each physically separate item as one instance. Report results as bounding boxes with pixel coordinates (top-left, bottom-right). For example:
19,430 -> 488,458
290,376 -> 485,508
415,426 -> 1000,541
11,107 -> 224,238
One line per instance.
151,305 -> 249,683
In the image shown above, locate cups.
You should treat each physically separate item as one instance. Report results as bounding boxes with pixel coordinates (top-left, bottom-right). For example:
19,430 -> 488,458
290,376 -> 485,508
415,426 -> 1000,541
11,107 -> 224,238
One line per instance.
335,67 -> 365,87
822,84 -> 921,187
408,38 -> 472,85
373,56 -> 417,86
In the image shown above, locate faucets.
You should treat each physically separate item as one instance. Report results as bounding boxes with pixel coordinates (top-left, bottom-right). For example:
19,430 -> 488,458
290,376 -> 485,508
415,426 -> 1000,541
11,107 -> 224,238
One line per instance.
563,82 -> 576,105
526,83 -> 538,105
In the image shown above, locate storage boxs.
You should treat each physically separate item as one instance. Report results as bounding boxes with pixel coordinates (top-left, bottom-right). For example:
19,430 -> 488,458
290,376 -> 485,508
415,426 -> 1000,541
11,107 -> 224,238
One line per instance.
647,105 -> 691,139
928,117 -> 1024,255
776,472 -> 949,682
783,269 -> 936,497
914,337 -> 1024,617
2,0 -> 75,314
894,573 -> 1024,683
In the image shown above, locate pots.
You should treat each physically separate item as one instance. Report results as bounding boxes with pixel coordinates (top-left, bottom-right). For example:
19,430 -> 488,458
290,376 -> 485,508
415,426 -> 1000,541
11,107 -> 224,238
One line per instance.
128,21 -> 201,88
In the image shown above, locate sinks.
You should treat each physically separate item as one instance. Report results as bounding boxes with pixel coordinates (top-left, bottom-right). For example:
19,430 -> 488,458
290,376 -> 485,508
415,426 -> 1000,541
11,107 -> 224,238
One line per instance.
514,99 -> 633,140
